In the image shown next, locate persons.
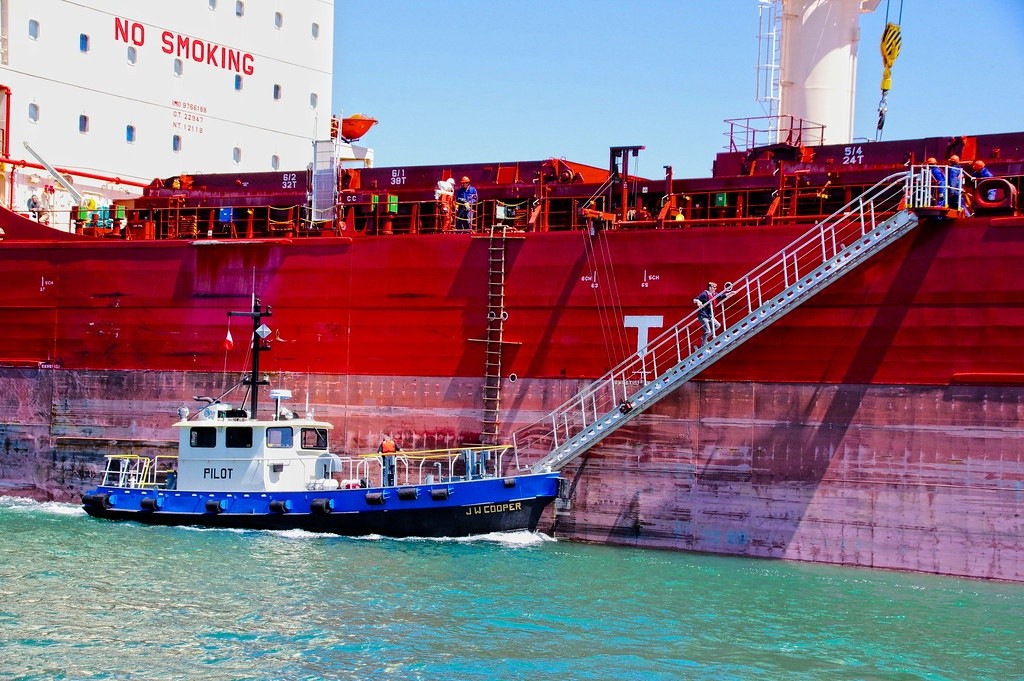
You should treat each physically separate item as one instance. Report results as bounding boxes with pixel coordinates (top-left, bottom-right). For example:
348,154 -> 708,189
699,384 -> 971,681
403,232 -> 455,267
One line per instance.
441,176 -> 478,234
377,435 -> 407,487
923,155 -> 970,220
692,282 -> 736,348
29,195 -> 49,223
973,161 -> 996,200
166,462 -> 177,490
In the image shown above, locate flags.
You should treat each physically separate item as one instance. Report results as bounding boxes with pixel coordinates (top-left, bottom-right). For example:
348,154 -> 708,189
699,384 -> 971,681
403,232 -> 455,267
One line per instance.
225,329 -> 233,352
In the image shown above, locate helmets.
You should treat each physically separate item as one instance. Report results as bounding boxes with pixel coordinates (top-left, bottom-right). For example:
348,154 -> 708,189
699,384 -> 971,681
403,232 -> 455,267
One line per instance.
461,176 -> 471,183
447,178 -> 455,185
927,157 -> 937,165
972,161 -> 985,170
948,155 -> 959,165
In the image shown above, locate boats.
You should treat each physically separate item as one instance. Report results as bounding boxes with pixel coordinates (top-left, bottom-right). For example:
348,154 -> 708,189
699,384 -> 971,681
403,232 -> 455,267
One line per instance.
76,295 -> 569,541
